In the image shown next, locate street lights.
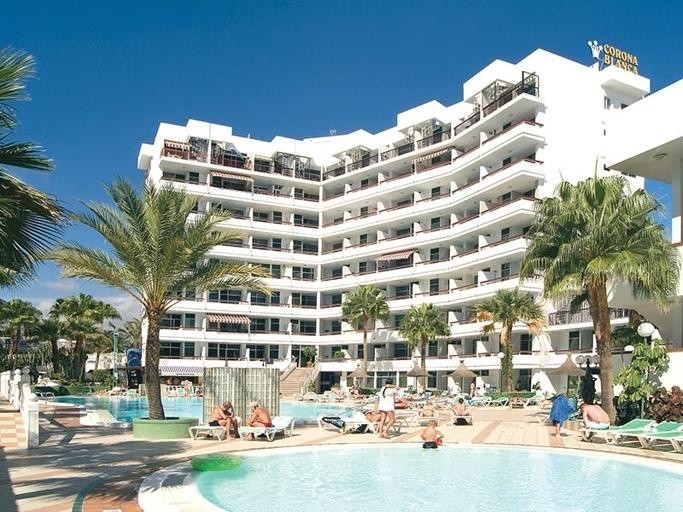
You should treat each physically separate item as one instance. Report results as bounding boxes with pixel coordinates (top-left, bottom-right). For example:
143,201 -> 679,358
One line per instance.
637,322 -> 654,346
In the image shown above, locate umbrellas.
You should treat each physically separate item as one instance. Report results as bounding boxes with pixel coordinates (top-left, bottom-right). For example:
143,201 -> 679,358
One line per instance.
402,363 -> 436,393
544,354 -> 586,397
441,360 -> 478,394
345,364 -> 370,387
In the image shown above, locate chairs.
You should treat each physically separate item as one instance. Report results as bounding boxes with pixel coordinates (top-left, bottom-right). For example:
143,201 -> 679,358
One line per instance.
543,390 -> 683,455
108,387 -> 140,397
238,415 -> 296,442
167,386 -> 210,398
301,389 -> 541,442
188,416 -> 243,442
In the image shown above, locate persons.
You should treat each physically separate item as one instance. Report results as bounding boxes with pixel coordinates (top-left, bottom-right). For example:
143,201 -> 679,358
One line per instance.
206,401 -> 241,442
242,400 -> 272,441
329,374 -> 611,449
